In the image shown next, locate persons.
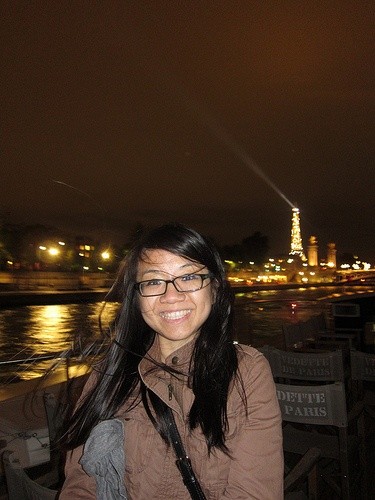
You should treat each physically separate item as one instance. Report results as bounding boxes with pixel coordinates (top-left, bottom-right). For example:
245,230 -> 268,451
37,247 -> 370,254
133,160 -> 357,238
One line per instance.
57,222 -> 286,499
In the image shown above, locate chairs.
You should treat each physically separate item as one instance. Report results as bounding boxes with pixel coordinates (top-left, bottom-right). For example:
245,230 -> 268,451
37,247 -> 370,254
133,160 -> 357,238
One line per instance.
261,327 -> 375,500
3,449 -> 60,500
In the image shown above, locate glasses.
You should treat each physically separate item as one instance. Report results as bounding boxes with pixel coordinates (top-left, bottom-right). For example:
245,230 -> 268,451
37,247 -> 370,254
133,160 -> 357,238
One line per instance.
134,273 -> 216,297
